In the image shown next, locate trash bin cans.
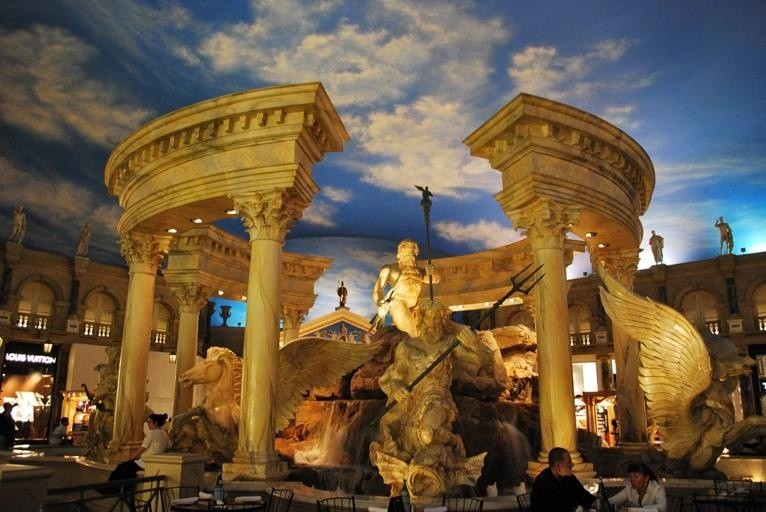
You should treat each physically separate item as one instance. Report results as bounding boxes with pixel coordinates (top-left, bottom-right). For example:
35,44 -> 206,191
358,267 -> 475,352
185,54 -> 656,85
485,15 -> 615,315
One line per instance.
33,406 -> 50,438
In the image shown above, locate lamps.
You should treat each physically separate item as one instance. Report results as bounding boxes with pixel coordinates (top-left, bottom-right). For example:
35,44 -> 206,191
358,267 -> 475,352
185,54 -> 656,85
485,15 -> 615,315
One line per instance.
43,337 -> 53,354
168,351 -> 177,363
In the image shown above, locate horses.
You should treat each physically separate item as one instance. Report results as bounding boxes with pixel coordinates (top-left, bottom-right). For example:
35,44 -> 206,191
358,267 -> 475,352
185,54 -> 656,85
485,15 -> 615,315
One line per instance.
160,346 -> 242,462
687,347 -> 766,473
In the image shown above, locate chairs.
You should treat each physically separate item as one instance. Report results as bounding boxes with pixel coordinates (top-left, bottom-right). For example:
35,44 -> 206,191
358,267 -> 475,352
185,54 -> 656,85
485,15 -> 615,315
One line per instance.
316,495 -> 356,511
516,492 -> 531,509
442,494 -> 484,510
666,476 -> 765,511
113,480 -> 295,511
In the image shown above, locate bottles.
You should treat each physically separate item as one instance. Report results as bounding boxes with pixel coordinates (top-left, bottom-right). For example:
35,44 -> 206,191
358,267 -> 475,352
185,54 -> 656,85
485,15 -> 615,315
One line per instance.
400,478 -> 410,512
213,471 -> 225,504
596,476 -> 609,512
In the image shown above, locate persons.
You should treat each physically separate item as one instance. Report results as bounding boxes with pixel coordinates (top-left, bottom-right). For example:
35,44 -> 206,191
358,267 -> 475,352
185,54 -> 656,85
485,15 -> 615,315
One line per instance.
9,205 -> 26,242
715,216 -> 732,254
378,299 -> 511,462
530,448 -> 597,512
82,346 -> 121,455
592,462 -> 668,511
0,401 -> 72,452
419,185 -> 433,208
77,223 -> 90,257
95,413 -> 172,496
371,239 -> 441,337
650,230 -> 665,263
314,325 -> 360,344
337,280 -> 348,306
